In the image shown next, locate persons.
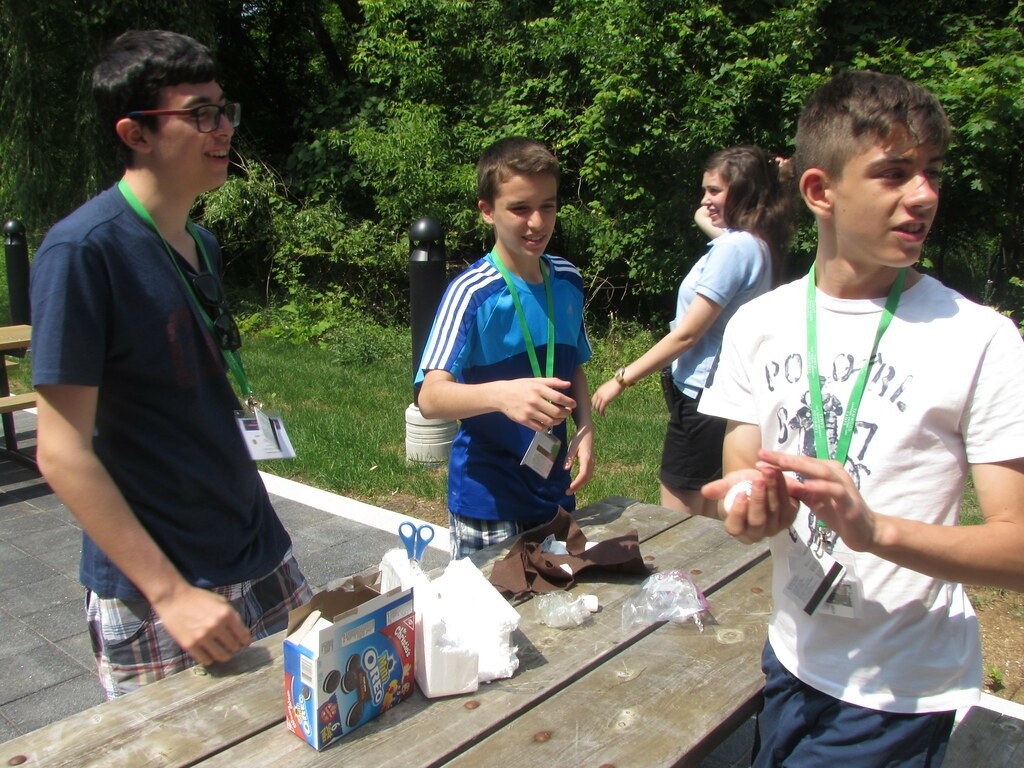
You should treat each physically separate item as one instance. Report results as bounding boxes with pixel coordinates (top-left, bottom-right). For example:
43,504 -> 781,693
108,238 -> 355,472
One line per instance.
590,146 -> 793,518
696,70 -> 1024,768
29,29 -> 313,701
412,134 -> 595,563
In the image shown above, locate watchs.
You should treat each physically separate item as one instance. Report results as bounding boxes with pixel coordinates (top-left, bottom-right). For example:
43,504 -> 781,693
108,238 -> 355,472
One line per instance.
615,367 -> 635,388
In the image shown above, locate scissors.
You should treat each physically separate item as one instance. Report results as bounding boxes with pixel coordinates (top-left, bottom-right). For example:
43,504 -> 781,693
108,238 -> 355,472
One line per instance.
398,522 -> 434,566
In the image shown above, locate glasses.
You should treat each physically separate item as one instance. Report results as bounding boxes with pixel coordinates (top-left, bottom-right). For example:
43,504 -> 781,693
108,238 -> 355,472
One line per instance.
127,101 -> 242,134
186,272 -> 242,355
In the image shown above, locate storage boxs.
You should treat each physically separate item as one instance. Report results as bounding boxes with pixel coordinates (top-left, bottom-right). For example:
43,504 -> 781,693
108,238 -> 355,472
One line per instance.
284,570 -> 415,752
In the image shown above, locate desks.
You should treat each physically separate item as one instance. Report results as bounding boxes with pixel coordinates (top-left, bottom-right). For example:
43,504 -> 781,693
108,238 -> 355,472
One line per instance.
0,324 -> 38,472
0,493 -> 774,768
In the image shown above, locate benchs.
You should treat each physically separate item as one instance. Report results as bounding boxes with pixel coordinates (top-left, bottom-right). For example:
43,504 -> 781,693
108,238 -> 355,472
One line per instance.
0,392 -> 37,414
